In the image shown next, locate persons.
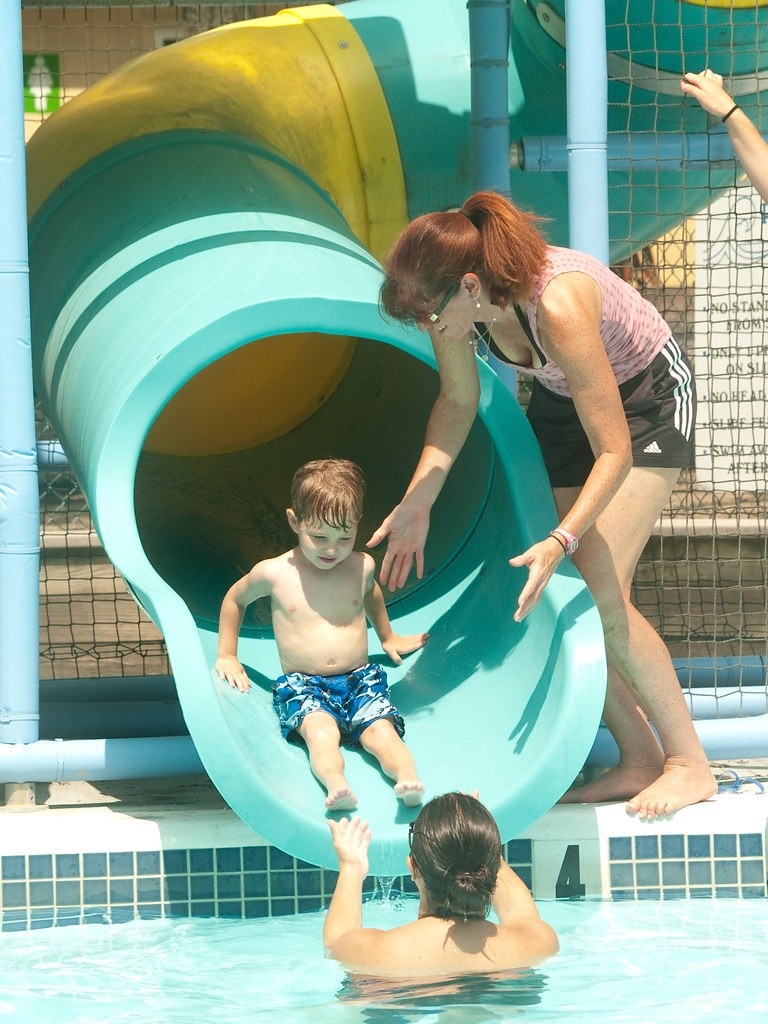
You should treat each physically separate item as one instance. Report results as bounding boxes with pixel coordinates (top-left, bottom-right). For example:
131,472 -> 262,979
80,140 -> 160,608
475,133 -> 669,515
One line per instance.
216,457 -> 432,811
323,787 -> 562,980
681,69 -> 768,208
361,189 -> 721,817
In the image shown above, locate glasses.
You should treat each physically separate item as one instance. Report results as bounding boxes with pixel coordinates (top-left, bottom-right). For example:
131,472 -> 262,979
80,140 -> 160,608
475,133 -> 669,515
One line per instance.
409,821 -> 416,849
410,279 -> 461,325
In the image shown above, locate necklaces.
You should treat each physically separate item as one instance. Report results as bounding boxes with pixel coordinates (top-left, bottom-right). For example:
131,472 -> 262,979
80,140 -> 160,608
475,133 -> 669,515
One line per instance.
471,312 -> 500,361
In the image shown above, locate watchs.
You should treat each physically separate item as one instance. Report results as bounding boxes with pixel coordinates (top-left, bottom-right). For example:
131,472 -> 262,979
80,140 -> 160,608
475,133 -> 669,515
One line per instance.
552,527 -> 578,555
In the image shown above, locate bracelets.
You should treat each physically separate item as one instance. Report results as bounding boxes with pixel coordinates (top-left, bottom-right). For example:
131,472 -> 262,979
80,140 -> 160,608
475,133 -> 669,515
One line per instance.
722,104 -> 739,125
548,535 -> 568,557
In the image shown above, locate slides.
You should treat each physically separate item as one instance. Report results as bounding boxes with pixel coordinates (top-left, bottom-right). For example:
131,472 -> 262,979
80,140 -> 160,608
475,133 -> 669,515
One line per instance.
24,0 -> 768,879
